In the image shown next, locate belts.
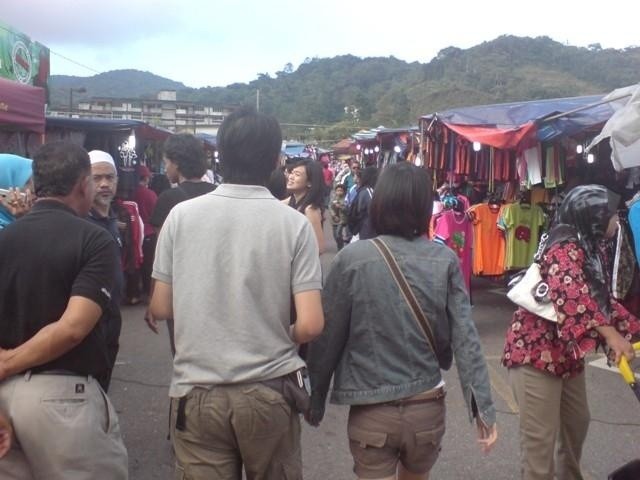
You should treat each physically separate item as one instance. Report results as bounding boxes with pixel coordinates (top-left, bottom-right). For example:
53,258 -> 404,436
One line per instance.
18,365 -> 95,378
376,390 -> 448,405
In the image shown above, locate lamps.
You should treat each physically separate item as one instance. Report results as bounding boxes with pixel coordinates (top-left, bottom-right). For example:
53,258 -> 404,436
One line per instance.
356,144 -> 380,155
587,153 -> 594,164
576,144 -> 583,154
214,150 -> 219,163
393,145 -> 401,153
472,141 -> 480,151
128,135 -> 136,149
415,153 -> 421,166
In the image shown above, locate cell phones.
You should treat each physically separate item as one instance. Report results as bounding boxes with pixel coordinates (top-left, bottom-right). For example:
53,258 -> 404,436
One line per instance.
0,189 -> 26,205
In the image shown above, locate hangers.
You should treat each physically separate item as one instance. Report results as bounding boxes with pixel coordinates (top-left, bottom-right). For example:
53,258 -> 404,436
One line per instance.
436,184 -> 531,212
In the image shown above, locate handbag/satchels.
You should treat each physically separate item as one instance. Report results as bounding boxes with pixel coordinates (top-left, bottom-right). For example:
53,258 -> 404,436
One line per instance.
505,260 -> 561,323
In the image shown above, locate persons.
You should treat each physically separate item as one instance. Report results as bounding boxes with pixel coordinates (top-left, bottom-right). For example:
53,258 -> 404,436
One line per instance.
85,133 -> 222,360
500,184 -> 640,480
0,139 -> 129,480
1,154 -> 36,228
304,161 -> 497,480
266,158 -> 380,378
145,108 -> 325,480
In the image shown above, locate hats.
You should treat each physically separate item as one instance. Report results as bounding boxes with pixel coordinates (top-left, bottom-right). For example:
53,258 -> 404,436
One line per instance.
87,149 -> 117,174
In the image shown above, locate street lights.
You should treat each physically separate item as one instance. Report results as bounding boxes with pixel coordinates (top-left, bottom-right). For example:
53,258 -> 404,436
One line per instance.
69,85 -> 87,118
